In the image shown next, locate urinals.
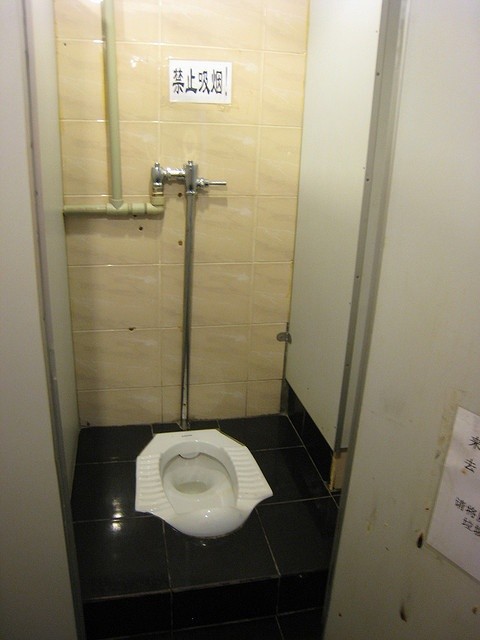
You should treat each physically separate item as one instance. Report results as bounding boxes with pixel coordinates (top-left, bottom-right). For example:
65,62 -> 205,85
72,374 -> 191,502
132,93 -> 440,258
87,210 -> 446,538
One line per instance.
134,428 -> 273,540
